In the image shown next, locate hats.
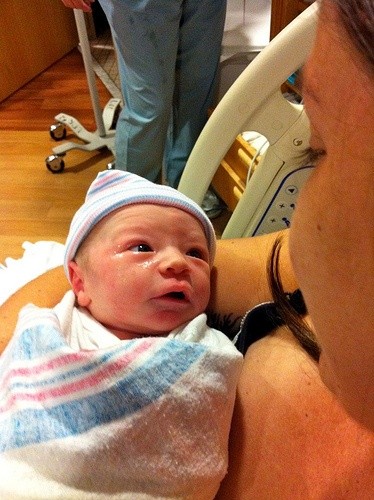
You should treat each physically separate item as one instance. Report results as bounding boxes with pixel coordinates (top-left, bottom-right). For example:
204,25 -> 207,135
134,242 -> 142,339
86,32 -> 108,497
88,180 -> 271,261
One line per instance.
63,169 -> 216,286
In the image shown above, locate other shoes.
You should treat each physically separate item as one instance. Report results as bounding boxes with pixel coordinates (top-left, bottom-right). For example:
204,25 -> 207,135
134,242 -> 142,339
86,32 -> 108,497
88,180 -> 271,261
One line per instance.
200,188 -> 222,219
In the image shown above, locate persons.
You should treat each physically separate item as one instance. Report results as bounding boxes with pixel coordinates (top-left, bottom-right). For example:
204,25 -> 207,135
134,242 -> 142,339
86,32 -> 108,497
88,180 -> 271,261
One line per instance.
61,0 -> 223,218
0,0 -> 374,500
0,170 -> 245,500
